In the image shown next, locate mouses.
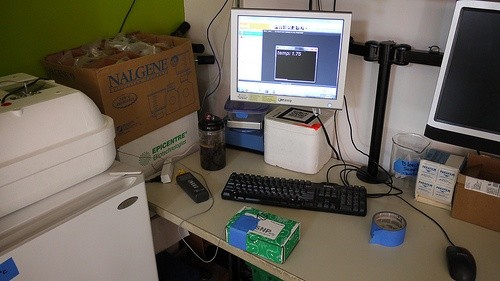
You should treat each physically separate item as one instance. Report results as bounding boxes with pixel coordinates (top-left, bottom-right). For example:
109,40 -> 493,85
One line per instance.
447,245 -> 476,281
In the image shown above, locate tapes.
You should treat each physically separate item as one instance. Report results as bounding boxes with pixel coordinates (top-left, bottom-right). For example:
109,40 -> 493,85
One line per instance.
370,211 -> 407,247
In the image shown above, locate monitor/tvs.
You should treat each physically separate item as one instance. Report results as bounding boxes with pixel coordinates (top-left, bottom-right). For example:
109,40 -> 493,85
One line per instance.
230,9 -> 352,109
424,0 -> 500,156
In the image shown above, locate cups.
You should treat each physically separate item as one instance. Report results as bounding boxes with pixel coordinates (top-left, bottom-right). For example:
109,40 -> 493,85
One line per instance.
389,133 -> 430,191
198,115 -> 226,171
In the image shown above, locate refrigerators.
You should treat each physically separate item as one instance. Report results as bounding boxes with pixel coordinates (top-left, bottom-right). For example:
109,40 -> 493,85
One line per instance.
0,161 -> 159,281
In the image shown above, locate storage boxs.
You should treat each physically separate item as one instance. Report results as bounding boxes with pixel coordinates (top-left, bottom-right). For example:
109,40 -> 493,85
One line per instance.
391,133 -> 431,195
116,111 -> 201,178
53,35 -> 199,145
226,207 -> 300,263
450,151 -> 500,232
265,107 -> 337,174
417,152 -> 465,209
224,94 -> 272,132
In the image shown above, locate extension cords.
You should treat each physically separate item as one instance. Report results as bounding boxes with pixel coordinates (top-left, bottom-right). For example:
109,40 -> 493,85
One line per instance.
161,163 -> 175,184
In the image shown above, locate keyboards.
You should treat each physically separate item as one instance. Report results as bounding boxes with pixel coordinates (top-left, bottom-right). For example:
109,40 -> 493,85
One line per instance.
222,172 -> 368,216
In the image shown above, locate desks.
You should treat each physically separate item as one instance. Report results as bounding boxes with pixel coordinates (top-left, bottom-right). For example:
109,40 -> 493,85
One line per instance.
147,148 -> 500,281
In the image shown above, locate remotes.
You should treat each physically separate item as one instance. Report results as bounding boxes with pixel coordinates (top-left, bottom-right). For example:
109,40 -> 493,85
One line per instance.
176,173 -> 208,203
